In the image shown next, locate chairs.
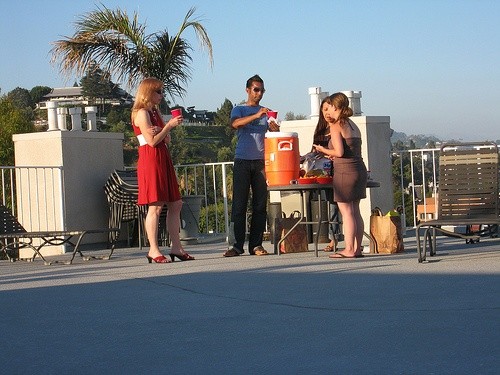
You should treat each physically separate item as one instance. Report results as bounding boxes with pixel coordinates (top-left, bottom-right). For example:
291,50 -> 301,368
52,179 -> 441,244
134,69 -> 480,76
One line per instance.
415,142 -> 500,263
0,203 -> 121,266
105,168 -> 170,250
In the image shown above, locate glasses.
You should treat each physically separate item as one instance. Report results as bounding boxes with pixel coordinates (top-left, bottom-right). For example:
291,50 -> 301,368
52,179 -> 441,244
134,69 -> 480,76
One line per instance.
252,87 -> 265,94
154,89 -> 166,94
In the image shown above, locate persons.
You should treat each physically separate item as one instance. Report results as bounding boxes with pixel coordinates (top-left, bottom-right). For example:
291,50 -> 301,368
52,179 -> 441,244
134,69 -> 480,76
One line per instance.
298,93 -> 368,258
130,77 -> 195,263
222,74 -> 280,257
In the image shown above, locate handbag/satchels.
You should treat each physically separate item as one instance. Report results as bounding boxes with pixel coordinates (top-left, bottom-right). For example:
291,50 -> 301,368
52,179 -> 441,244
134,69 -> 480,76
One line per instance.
276,210 -> 309,253
370,206 -> 405,254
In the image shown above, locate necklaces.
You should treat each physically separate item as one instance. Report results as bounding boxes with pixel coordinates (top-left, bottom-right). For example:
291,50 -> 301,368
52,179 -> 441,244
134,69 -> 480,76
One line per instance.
150,108 -> 156,117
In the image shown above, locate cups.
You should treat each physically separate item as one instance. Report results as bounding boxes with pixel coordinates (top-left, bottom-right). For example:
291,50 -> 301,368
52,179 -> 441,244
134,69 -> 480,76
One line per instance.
170,108 -> 181,118
267,111 -> 278,120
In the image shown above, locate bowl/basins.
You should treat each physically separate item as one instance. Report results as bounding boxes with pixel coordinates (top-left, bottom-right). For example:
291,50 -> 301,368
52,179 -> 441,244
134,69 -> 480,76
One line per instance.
317,177 -> 333,184
297,178 -> 316,184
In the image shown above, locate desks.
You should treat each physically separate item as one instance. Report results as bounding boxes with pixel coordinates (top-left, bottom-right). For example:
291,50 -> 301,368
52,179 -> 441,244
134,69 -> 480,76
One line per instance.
267,180 -> 381,258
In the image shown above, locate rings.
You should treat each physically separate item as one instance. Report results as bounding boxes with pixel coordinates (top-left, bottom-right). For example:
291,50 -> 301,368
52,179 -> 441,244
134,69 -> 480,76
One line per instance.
152,130 -> 156,133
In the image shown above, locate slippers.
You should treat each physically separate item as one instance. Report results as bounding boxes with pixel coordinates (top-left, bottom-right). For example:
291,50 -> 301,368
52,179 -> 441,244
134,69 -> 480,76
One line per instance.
223,246 -> 244,256
324,246 -> 338,252
250,246 -> 269,255
330,253 -> 365,258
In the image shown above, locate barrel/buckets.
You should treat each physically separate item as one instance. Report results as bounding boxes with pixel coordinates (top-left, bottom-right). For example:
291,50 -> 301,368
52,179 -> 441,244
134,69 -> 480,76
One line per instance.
264,132 -> 300,186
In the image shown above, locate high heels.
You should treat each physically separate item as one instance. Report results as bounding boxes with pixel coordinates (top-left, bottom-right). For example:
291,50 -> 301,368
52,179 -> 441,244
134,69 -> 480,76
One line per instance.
147,253 -> 170,264
169,253 -> 195,262
